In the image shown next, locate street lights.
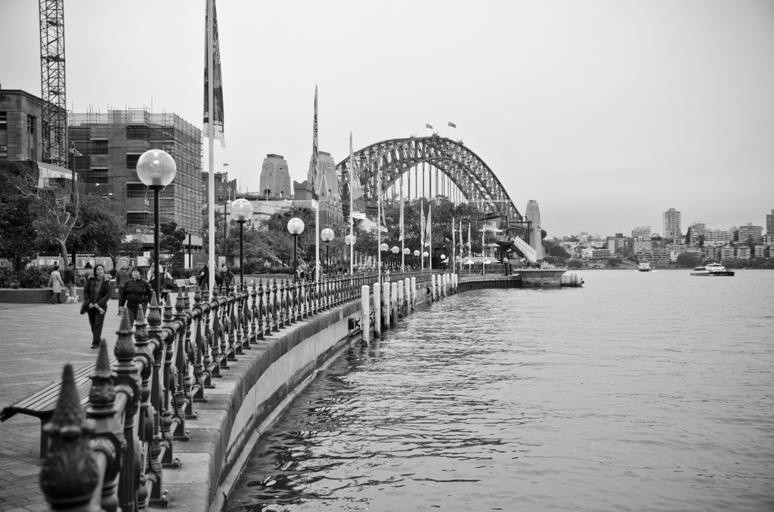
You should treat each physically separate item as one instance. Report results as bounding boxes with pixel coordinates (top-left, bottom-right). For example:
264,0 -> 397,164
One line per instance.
286,217 -> 446,283
229,198 -> 252,294
135,148 -> 177,306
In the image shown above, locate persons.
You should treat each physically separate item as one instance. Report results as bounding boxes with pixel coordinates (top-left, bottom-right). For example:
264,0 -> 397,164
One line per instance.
48,261 -> 234,329
297,264 -> 422,282
81,264 -> 112,349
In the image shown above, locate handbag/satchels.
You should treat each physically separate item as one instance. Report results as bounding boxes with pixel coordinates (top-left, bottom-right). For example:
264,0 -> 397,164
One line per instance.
80,304 -> 87,314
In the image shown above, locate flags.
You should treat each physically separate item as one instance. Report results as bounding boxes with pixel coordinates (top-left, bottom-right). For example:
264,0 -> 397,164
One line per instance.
448,120 -> 456,128
426,123 -> 434,129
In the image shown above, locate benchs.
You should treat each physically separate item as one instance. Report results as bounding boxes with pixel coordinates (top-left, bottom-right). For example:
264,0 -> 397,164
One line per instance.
0,356 -> 120,460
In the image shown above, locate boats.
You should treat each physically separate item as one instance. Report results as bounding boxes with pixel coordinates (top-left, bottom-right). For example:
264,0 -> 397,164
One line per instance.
690,262 -> 734,276
638,263 -> 651,271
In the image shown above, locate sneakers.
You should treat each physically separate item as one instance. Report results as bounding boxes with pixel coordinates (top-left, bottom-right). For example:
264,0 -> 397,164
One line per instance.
91,344 -> 98,348
